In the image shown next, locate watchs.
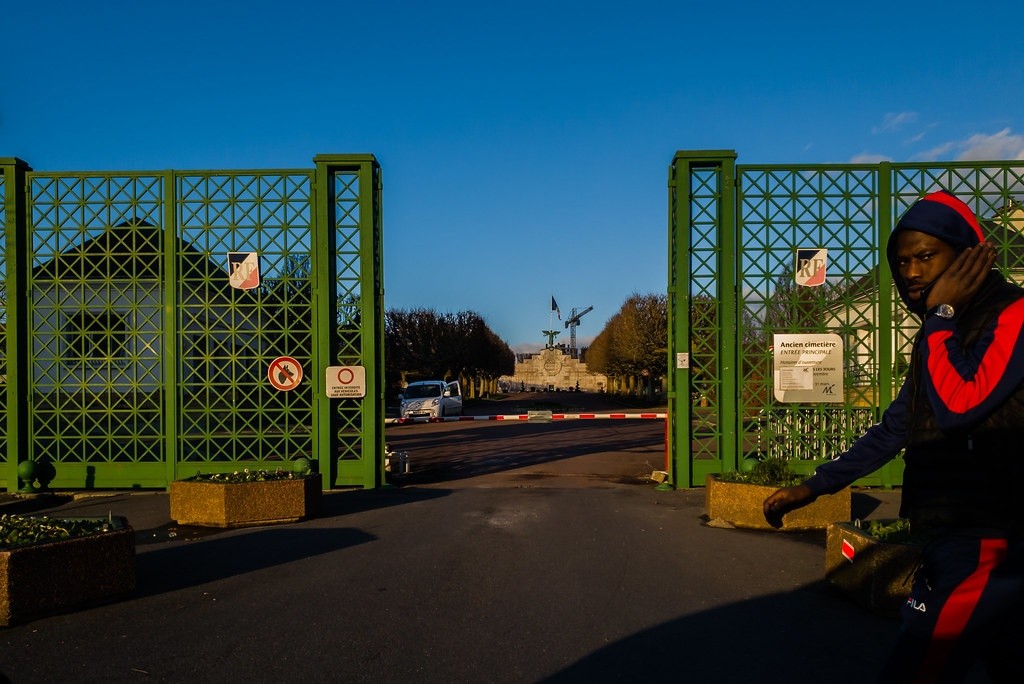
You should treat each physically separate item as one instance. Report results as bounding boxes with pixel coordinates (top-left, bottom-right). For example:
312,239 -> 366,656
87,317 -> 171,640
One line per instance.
925,304 -> 955,320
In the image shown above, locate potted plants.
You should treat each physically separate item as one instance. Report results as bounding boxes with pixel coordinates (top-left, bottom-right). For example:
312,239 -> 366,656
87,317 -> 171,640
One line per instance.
824,517 -> 916,597
171,471 -> 323,528
705,459 -> 852,533
0,512 -> 136,627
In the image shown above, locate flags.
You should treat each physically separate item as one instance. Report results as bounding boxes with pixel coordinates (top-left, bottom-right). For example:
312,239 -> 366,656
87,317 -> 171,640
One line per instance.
552,296 -> 562,321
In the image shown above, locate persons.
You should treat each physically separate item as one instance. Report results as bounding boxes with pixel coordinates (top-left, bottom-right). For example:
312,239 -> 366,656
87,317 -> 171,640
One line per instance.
764,190 -> 1024,684
543,330 -> 557,348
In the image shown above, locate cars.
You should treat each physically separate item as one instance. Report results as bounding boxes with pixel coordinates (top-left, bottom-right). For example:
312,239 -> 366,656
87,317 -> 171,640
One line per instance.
397,380 -> 463,417
